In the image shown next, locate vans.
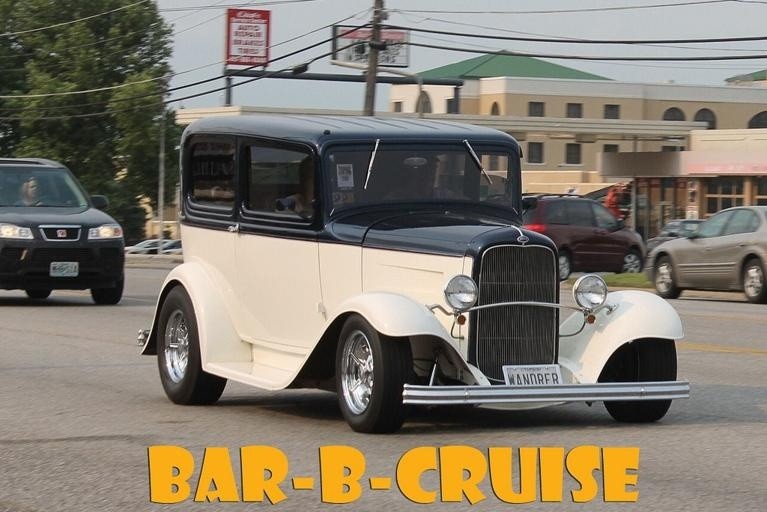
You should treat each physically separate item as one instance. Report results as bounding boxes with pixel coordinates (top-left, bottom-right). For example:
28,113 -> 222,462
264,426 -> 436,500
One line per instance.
514,192 -> 648,283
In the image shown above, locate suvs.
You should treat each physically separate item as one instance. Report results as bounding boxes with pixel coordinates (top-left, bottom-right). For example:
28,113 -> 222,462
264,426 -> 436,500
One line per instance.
0,156 -> 127,307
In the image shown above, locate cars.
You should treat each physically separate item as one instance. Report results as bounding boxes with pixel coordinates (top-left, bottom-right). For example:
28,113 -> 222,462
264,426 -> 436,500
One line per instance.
642,204 -> 767,303
133,113 -> 692,435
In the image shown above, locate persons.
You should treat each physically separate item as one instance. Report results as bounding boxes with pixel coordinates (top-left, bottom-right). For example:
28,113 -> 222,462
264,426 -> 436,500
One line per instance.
606,189 -> 620,222
275,156 -> 315,213
391,158 -> 455,200
13,177 -> 47,207
